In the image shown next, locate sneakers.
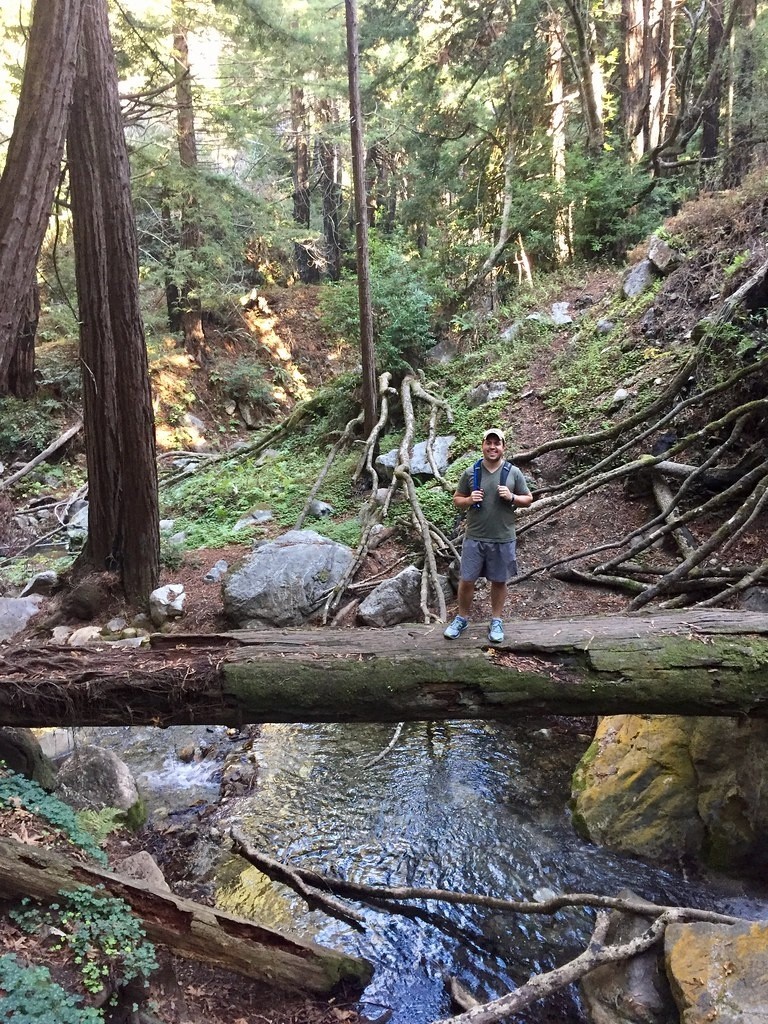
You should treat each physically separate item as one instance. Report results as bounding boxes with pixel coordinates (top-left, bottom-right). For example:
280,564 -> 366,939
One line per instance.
443,615 -> 468,640
487,618 -> 504,642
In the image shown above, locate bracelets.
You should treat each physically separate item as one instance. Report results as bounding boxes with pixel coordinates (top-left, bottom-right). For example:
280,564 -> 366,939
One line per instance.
510,492 -> 514,502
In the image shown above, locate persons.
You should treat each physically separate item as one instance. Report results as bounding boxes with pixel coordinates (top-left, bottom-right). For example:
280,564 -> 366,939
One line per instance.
442,428 -> 534,642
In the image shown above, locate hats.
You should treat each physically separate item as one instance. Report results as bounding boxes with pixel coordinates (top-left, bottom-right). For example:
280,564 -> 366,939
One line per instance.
483,428 -> 505,441
